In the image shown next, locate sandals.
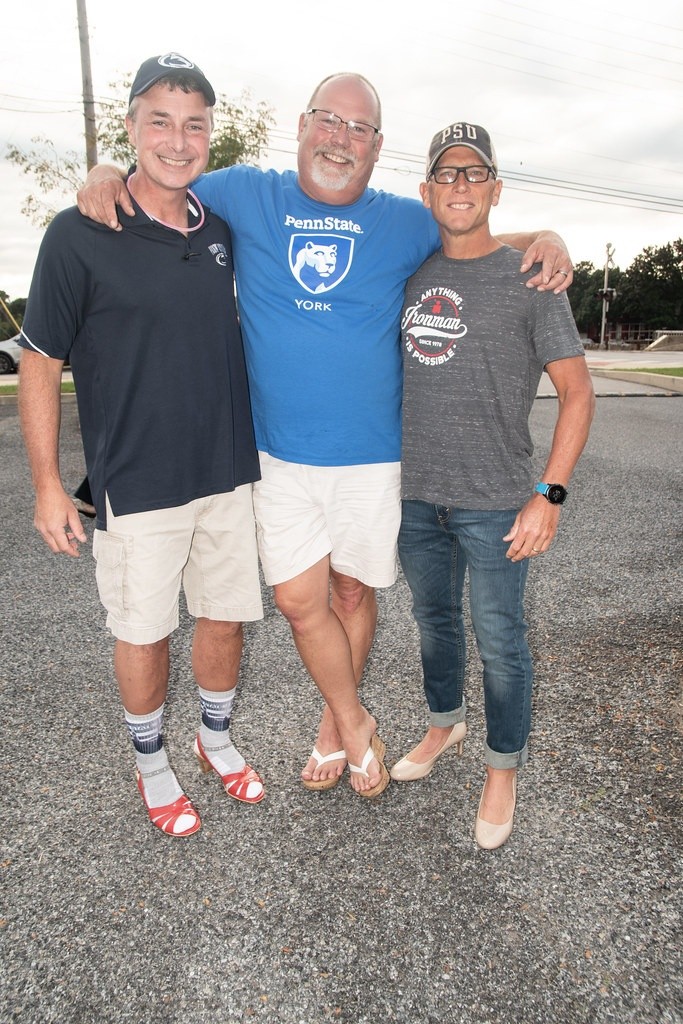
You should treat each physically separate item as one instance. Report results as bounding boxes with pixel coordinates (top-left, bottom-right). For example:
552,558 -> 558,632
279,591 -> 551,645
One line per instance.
135,764 -> 201,837
193,733 -> 265,804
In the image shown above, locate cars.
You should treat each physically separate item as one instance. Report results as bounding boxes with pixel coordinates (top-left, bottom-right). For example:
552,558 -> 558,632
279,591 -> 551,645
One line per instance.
0,333 -> 25,374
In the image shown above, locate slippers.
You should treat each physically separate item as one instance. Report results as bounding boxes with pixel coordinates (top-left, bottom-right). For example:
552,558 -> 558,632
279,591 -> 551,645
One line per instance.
301,731 -> 390,799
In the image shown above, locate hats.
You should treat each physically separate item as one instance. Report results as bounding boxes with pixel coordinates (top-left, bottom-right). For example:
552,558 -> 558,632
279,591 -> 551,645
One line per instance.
129,52 -> 215,106
426,122 -> 498,181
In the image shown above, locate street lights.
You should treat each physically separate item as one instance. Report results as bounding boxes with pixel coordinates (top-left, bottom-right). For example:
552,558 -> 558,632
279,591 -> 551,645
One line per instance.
599,241 -> 616,348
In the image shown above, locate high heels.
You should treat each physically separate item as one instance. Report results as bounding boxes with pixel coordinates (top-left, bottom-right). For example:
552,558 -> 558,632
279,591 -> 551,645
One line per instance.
475,770 -> 517,850
389,722 -> 466,781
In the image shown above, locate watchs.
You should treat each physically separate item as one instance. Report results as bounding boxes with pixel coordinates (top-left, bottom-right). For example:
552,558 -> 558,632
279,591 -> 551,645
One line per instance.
535,482 -> 568,507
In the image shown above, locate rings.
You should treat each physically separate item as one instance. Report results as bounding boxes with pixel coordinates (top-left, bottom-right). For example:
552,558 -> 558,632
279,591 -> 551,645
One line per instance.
556,270 -> 567,278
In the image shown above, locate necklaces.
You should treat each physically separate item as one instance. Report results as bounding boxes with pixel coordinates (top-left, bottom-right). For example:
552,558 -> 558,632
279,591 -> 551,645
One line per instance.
126,172 -> 204,232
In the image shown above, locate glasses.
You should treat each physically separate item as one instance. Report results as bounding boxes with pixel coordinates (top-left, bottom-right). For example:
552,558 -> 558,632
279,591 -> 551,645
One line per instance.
431,165 -> 496,184
307,108 -> 379,142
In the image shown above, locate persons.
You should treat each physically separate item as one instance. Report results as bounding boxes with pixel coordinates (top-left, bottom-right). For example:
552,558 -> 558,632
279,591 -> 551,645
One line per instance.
19,54 -> 262,837
389,124 -> 597,850
79,76 -> 575,797
